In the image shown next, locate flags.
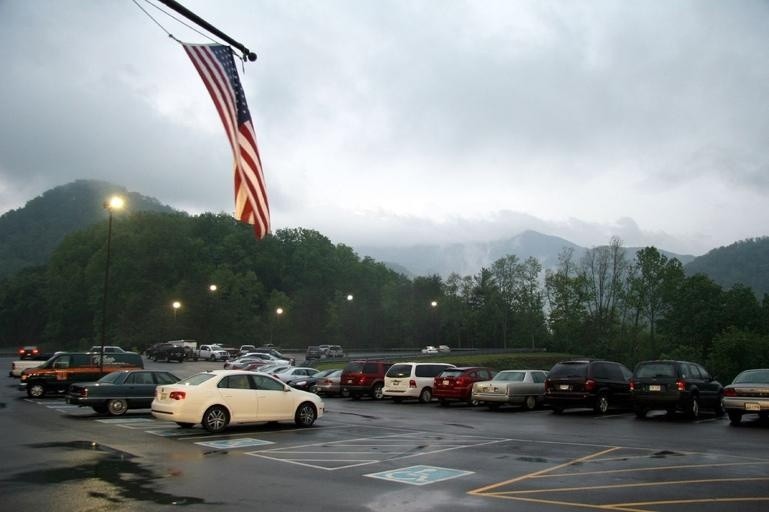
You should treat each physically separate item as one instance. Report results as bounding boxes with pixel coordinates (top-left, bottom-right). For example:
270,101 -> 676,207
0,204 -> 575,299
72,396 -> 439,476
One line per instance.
180,42 -> 273,239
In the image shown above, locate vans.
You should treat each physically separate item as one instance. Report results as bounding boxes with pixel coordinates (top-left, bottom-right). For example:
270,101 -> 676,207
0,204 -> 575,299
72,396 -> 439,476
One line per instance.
382,361 -> 457,403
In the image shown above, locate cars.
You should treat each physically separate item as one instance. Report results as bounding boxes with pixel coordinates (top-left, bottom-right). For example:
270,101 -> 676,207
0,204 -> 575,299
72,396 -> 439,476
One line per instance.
720,367 -> 769,426
421,346 -> 437,356
471,366 -> 549,411
151,368 -> 329,433
438,344 -> 451,354
62,369 -> 182,416
143,336 -> 345,394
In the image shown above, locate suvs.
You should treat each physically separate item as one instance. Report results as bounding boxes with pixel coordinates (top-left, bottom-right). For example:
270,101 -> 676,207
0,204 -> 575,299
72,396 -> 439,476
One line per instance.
544,357 -> 635,414
340,359 -> 397,401
627,359 -> 724,420
431,365 -> 498,409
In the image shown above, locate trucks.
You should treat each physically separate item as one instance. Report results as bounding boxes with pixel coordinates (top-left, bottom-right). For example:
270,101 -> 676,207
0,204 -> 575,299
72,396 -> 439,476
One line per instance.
11,345 -> 142,398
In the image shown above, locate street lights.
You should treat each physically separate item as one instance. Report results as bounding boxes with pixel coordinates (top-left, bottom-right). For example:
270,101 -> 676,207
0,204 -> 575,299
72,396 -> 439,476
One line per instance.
430,300 -> 438,346
209,284 -> 220,305
172,300 -> 182,335
100,192 -> 128,379
276,308 -> 283,323
